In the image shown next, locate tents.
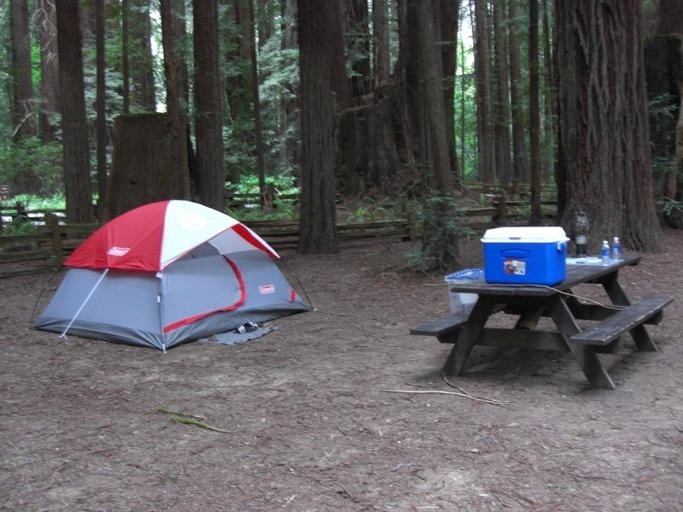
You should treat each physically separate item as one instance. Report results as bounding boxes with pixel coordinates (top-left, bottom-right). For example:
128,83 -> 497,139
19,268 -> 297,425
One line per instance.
29,197 -> 313,358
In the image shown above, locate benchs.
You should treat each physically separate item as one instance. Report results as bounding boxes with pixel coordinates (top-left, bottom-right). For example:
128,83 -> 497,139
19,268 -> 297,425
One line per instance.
565,296 -> 674,389
407,299 -> 510,379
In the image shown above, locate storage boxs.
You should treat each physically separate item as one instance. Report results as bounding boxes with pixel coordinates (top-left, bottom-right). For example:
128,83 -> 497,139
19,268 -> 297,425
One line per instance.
443,268 -> 484,314
479,224 -> 573,287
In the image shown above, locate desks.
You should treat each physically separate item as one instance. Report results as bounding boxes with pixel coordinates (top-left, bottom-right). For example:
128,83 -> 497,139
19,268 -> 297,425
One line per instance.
439,252 -> 660,390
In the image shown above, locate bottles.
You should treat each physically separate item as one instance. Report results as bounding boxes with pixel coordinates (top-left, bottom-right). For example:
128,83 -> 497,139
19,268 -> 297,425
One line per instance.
575,210 -> 588,262
611,236 -> 620,259
600,240 -> 610,266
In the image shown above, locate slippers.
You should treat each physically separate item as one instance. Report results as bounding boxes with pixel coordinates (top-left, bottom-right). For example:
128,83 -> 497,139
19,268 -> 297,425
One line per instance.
234,321 -> 262,334
